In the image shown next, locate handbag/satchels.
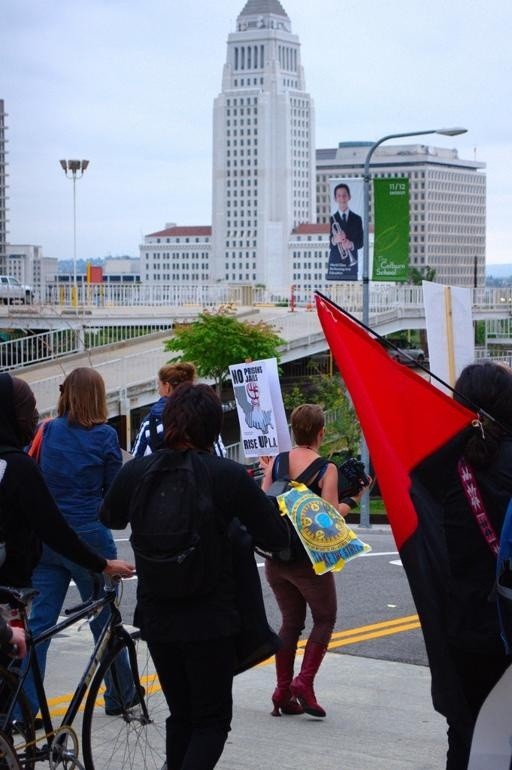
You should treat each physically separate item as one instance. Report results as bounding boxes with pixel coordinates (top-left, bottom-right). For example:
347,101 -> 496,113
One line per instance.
339,458 -> 369,498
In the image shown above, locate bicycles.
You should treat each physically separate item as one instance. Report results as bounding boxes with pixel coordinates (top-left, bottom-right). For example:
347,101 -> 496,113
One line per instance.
0,567 -> 175,770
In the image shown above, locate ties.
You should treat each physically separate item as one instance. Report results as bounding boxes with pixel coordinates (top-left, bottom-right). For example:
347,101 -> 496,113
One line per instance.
343,214 -> 346,222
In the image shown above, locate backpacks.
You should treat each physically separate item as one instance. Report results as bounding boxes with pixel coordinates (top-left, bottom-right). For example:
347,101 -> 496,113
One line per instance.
130,448 -> 223,600
252,453 -> 328,565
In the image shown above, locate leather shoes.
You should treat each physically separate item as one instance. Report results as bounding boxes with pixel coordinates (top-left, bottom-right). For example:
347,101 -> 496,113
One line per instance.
10,718 -> 43,734
106,686 -> 145,715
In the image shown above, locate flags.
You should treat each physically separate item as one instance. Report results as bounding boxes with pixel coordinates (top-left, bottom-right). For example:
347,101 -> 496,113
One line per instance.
313,295 -> 507,709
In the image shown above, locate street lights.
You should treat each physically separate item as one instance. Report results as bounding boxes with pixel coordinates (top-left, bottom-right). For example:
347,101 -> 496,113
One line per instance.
62,158 -> 88,316
360,126 -> 469,529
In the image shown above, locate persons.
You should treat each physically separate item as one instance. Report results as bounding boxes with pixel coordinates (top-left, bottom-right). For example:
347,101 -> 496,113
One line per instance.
130,364 -> 225,460
98,382 -> 289,770
323,183 -> 363,279
440,361 -> 512,768
1,374 -> 135,746
12,368 -> 145,731
260,405 -> 373,715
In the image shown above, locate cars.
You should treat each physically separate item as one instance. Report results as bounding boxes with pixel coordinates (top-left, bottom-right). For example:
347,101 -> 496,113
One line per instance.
376,338 -> 425,365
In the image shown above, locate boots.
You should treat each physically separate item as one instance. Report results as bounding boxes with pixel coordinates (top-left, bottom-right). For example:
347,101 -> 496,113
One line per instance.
271,651 -> 304,716
288,640 -> 327,716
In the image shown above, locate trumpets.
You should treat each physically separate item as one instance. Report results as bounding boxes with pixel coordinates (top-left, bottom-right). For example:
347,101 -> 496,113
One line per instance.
330,211 -> 357,267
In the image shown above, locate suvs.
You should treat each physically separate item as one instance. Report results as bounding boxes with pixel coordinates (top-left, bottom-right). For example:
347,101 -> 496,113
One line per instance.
0,276 -> 34,305
